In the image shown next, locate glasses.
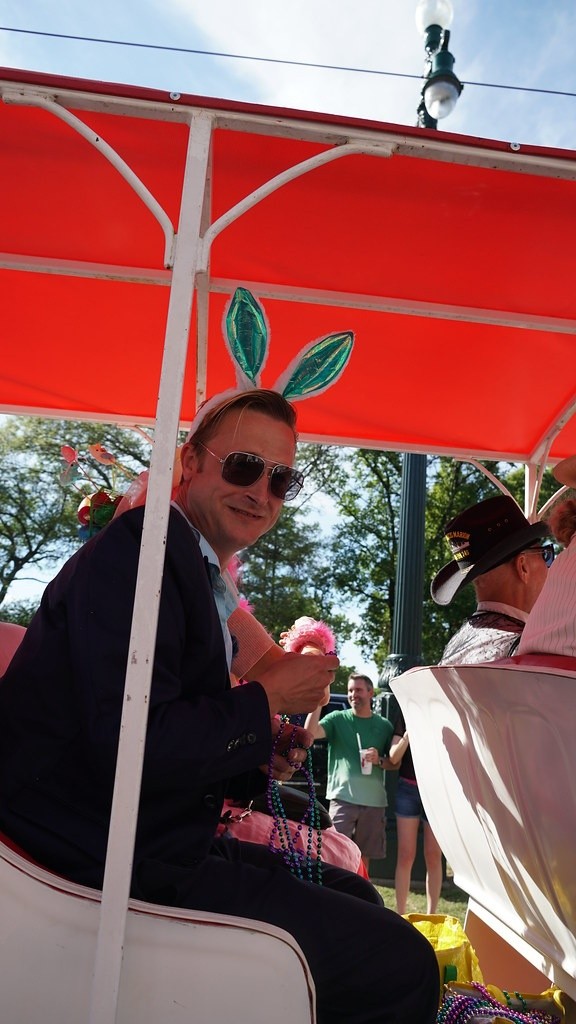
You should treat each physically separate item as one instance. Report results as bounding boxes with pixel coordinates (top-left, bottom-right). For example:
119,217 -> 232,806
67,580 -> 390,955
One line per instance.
522,544 -> 555,567
195,440 -> 306,501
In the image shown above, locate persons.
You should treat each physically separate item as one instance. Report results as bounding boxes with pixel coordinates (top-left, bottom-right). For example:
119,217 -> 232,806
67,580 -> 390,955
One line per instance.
389,711 -> 444,916
436,457 -> 576,668
1,387 -> 439,1024
304,676 -> 395,880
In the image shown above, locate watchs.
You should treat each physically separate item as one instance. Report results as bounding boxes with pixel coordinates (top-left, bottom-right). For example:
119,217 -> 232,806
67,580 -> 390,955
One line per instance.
377,756 -> 384,766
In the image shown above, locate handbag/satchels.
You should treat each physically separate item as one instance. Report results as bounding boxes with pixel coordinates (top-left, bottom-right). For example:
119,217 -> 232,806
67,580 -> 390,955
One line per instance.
404,913 -> 482,983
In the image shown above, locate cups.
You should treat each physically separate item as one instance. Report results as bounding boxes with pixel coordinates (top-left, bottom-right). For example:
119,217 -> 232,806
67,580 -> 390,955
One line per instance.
359,749 -> 374,775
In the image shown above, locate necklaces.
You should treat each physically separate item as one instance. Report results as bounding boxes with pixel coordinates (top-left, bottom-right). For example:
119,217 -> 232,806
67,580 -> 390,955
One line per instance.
267,650 -> 338,885
437,979 -> 563,1024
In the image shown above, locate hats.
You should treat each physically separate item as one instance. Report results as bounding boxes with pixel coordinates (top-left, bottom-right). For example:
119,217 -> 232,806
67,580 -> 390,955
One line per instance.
430,495 -> 549,606
553,455 -> 576,489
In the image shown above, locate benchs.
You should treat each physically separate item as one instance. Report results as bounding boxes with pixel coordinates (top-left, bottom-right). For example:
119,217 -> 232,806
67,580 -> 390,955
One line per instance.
0,624 -> 317,1024
388,653 -> 576,1001
